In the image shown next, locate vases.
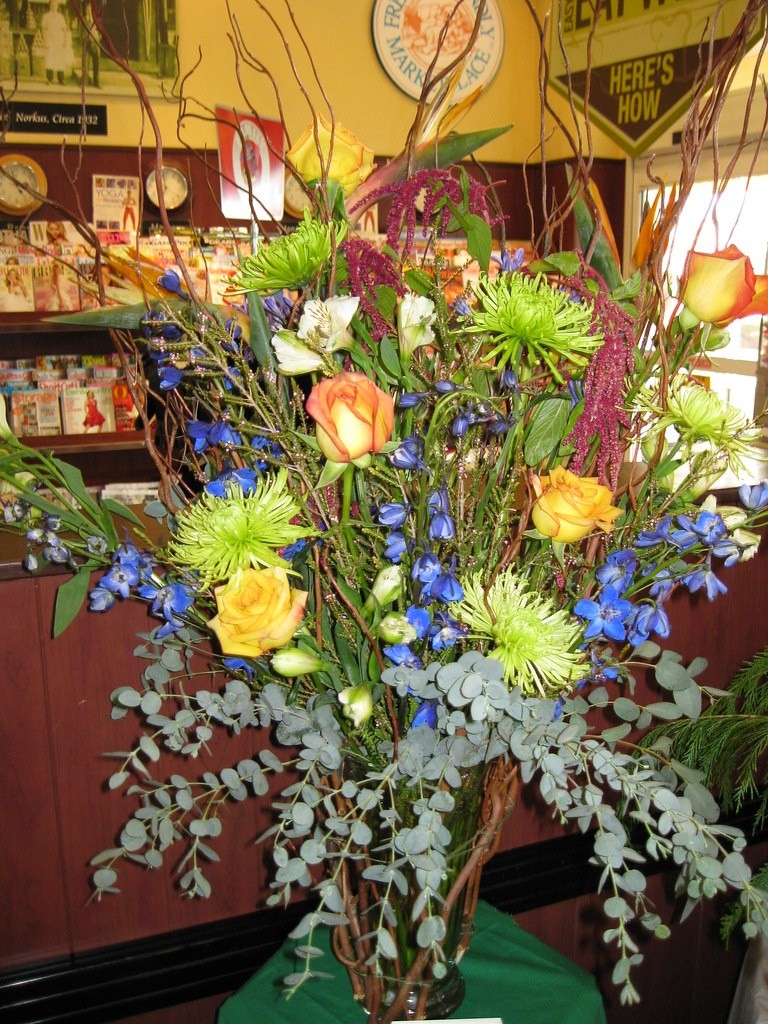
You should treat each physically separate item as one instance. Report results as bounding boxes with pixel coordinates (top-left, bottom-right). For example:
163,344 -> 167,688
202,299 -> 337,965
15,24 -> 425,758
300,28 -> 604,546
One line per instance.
345,755 -> 487,1024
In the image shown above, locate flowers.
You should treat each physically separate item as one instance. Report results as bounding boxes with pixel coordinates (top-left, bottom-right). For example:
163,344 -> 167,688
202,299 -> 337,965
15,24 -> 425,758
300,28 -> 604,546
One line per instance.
0,0 -> 768,1023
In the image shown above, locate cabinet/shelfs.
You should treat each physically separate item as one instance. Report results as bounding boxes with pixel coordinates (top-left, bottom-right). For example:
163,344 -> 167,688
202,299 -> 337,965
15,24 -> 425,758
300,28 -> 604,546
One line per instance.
1,312 -> 157,456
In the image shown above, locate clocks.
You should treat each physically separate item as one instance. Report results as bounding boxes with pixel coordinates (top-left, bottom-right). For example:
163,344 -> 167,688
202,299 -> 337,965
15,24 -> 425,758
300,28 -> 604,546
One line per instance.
140,157 -> 191,217
0,153 -> 48,217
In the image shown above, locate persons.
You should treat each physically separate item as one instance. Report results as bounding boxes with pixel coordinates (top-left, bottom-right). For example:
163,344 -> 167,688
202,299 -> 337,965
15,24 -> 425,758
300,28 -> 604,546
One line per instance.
3,269 -> 32,311
7,257 -> 18,265
46,265 -> 73,310
46,221 -> 67,243
123,190 -> 136,230
41,0 -> 70,85
80,5 -> 103,89
83,390 -> 105,433
34,245 -> 88,256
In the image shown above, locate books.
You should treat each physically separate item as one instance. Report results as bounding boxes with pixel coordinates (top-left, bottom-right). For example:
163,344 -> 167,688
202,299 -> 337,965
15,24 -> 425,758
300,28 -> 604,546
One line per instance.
0,173 -> 278,441
